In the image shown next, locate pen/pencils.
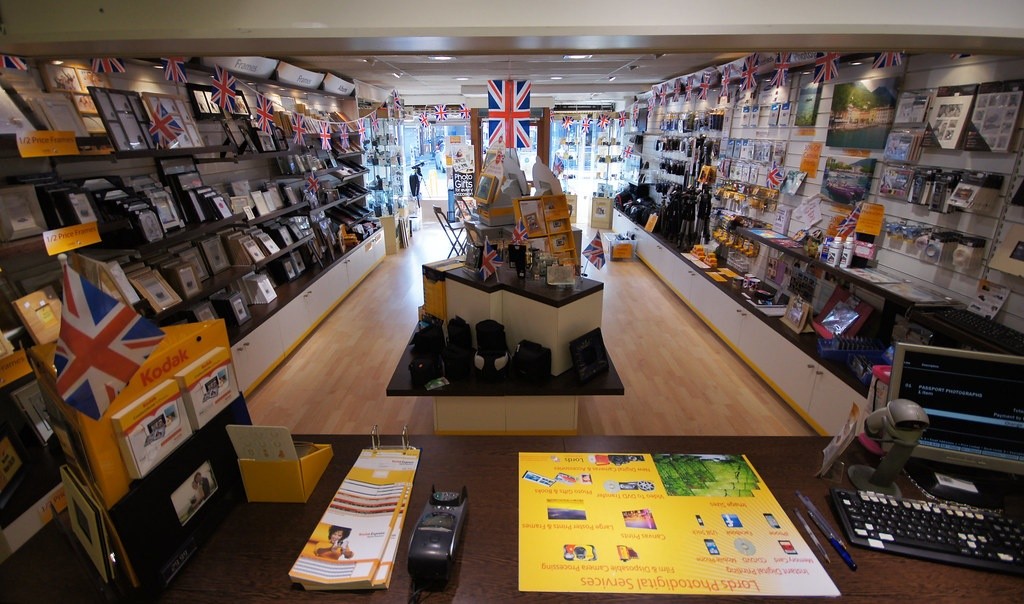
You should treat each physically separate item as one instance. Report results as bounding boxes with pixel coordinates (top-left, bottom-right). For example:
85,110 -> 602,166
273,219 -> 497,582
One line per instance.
793,508 -> 831,563
808,511 -> 857,571
804,495 -> 847,550
795,490 -> 837,541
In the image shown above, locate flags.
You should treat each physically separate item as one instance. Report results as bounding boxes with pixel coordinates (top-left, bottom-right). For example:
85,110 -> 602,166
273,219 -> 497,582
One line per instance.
0,54 -> 27,70
550,71 -> 711,132
836,205 -> 860,234
292,113 -> 307,147
487,79 -> 531,148
553,155 -> 564,172
512,220 -> 528,242
339,112 -> 380,147
91,57 -> 125,73
766,162 -> 782,189
256,94 -> 273,135
305,173 -> 321,195
582,230 -> 606,270
161,57 -> 187,82
720,53 -> 904,98
394,92 -> 471,127
149,103 -> 184,148
211,64 -> 237,113
54,263 -> 166,420
482,239 -> 503,282
624,147 -> 633,156
320,122 -> 332,151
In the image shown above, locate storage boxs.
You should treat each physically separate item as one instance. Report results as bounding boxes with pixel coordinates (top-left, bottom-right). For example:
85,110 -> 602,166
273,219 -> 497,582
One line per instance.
236,444 -> 333,502
604,233 -> 637,261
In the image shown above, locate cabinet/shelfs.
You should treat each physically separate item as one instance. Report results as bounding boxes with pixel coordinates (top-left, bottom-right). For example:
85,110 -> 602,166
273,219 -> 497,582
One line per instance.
0,137 -> 386,562
611,206 -> 1014,436
379,207 -> 410,255
422,191 -> 604,379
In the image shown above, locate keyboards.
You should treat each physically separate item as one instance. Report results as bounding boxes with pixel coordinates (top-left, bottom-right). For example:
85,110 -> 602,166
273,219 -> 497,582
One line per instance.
829,487 -> 1024,577
934,309 -> 1024,356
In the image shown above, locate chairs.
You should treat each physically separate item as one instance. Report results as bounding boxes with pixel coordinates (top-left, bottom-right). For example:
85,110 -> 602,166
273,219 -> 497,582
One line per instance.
432,195 -> 473,259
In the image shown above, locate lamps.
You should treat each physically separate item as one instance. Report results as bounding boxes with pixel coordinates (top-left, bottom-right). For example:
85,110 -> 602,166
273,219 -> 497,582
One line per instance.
653,54 -> 666,58
607,76 -> 614,80
627,66 -> 638,71
366,58 -> 378,66
392,73 -> 404,78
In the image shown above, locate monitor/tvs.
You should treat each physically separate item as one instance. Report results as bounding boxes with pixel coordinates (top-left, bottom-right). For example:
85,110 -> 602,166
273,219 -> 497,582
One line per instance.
882,342 -> 1024,512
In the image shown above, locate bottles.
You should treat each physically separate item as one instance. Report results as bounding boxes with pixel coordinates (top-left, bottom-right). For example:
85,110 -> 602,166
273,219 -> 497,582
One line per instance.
839,237 -> 855,270
819,235 -> 833,263
826,236 -> 843,268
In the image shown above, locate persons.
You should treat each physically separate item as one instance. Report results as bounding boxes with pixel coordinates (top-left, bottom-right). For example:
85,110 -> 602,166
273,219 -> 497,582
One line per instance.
315,525 -> 355,559
192,473 -> 210,507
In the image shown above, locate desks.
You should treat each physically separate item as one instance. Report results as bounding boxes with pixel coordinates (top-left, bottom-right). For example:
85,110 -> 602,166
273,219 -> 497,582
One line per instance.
0,434 -> 1024,604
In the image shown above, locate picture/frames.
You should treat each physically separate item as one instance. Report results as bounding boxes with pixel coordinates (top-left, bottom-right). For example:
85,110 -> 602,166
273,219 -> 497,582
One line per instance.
0,80 -> 374,510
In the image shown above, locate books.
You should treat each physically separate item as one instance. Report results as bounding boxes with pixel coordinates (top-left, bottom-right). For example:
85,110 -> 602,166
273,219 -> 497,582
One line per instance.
61,464 -> 115,584
